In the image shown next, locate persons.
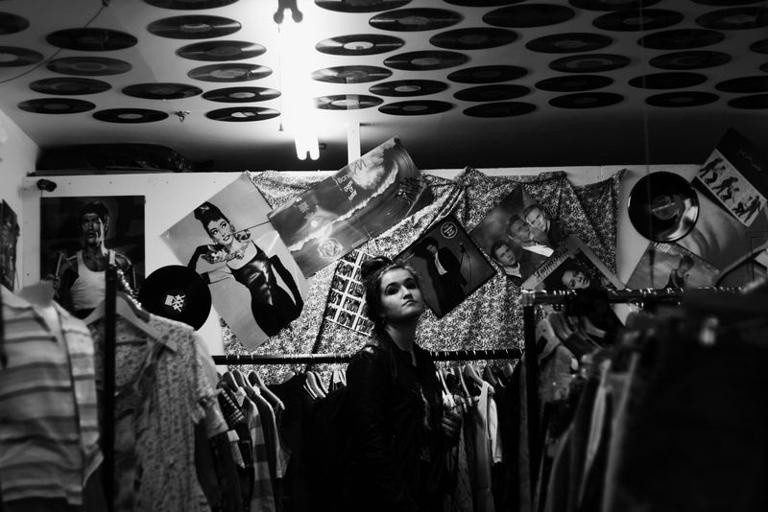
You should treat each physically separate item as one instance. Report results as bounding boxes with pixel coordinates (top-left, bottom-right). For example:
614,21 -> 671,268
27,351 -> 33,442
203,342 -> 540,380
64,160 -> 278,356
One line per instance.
699,156 -> 762,223
490,205 -> 619,291
54,202 -> 135,318
346,255 -> 446,512
188,201 -> 303,336
423,237 -> 466,314
664,255 -> 694,289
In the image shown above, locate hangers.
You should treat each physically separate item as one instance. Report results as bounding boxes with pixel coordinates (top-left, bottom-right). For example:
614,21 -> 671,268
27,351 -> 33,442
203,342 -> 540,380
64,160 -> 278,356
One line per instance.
427,346 -> 514,416
520,288 -> 578,364
222,350 -> 286,412
72,285 -> 179,356
302,350 -> 349,401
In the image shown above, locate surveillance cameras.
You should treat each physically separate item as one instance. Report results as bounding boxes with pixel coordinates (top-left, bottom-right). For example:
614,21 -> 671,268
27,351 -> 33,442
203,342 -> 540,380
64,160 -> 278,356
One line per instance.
37,179 -> 56,191
